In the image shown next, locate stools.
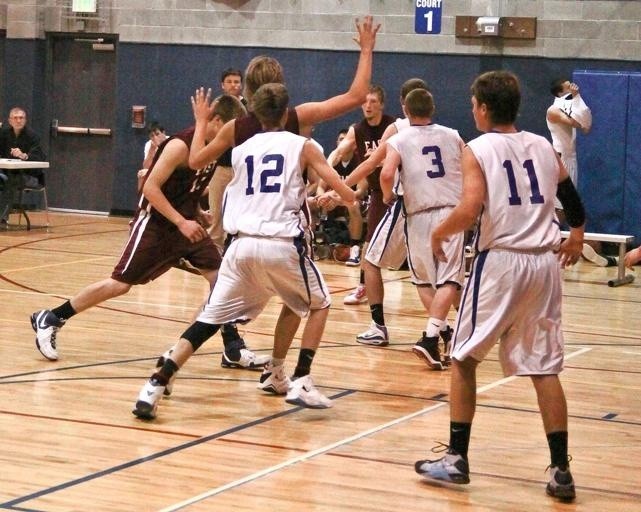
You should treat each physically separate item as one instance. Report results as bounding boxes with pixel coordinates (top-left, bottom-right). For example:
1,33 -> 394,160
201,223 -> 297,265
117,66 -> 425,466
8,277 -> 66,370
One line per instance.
12,185 -> 49,228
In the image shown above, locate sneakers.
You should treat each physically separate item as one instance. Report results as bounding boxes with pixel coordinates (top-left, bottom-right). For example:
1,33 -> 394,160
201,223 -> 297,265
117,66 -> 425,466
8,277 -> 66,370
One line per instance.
345,245 -> 360,265
30,309 -> 66,361
415,453 -> 470,485
545,466 -> 576,499
581,244 -> 609,267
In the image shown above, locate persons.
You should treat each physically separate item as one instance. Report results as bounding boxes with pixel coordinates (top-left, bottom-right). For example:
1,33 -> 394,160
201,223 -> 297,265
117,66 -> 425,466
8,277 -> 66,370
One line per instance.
129,120 -> 169,226
0,107 -> 48,229
299,78 -> 467,372
206,67 -> 247,255
187,14 -> 382,395
130,80 -> 357,421
545,79 -> 593,227
30,93 -> 272,369
580,243 -> 641,268
415,69 -> 585,504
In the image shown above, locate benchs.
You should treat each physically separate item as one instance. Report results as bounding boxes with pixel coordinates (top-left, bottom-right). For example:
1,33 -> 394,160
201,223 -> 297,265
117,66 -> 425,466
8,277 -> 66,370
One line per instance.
560,230 -> 634,288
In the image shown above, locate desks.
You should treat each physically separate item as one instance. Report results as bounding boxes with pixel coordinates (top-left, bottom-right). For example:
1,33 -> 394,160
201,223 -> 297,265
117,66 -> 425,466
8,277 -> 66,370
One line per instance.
1,158 -> 49,231
319,216 -> 368,233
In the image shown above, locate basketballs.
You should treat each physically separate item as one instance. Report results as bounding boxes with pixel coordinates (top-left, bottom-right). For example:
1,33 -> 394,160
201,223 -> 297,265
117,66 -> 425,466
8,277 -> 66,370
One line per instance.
333,243 -> 351,261
